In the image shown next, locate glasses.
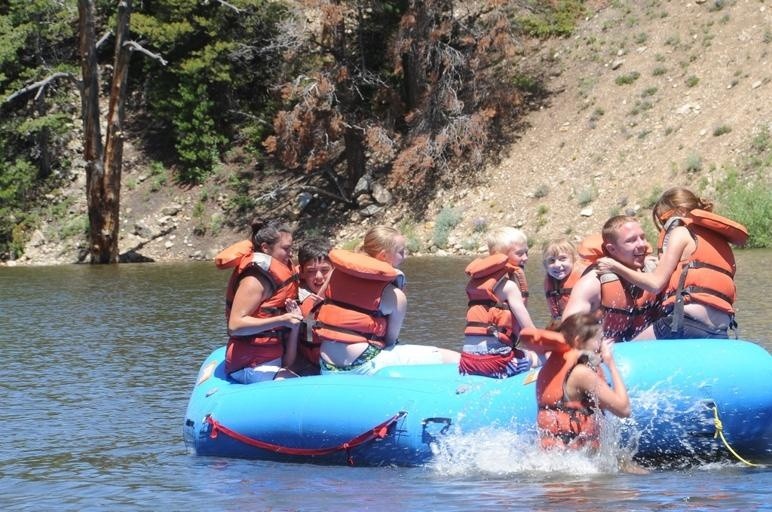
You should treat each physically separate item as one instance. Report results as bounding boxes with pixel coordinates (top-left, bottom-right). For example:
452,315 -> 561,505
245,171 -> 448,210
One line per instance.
659,207 -> 677,225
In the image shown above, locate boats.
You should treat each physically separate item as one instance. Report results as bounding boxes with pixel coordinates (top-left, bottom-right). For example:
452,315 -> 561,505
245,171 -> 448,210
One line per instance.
182,336 -> 772,477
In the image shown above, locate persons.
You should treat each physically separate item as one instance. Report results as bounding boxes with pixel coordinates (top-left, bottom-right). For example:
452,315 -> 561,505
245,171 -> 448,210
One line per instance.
561,215 -> 660,342
542,240 -> 584,328
520,311 -> 631,455
596,188 -> 749,341
214,216 -> 304,383
285,237 -> 334,369
315,226 -> 409,372
459,225 -> 547,380
